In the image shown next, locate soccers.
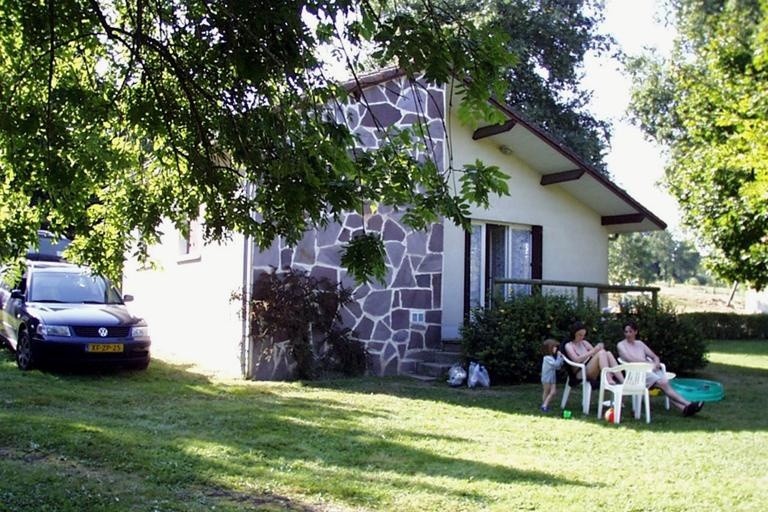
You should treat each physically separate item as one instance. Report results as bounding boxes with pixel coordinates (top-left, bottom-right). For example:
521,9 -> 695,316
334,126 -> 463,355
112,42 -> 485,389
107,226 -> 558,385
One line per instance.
604,408 -> 614,423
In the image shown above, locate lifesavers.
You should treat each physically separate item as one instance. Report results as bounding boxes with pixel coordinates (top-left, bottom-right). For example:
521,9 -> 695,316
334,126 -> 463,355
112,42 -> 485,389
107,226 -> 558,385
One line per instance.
668,379 -> 724,400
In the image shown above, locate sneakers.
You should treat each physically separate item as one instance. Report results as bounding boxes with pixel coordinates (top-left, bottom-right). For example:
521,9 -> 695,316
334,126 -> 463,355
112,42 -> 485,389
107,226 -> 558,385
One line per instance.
539,407 -> 552,413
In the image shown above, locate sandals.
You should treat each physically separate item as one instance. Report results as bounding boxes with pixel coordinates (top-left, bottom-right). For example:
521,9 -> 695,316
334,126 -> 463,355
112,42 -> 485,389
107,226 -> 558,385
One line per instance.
682,399 -> 704,416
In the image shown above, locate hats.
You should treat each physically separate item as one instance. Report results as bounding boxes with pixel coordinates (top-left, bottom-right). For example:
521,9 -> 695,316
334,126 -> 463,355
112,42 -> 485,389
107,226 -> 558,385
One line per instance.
542,339 -> 561,355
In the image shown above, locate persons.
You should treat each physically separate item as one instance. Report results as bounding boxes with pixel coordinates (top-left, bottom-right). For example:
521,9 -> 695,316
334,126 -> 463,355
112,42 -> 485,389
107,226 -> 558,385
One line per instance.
564,321 -> 627,385
616,322 -> 705,418
539,339 -> 565,413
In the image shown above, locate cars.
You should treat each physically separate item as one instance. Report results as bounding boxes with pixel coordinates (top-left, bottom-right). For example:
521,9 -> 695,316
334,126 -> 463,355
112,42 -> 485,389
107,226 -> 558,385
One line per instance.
620,292 -> 651,312
0,229 -> 152,370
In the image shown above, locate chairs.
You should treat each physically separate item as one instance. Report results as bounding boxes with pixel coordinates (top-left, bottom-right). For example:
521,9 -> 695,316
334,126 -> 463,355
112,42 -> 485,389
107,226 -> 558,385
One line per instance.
597,363 -> 654,424
560,353 -> 616,415
618,357 -> 670,411
